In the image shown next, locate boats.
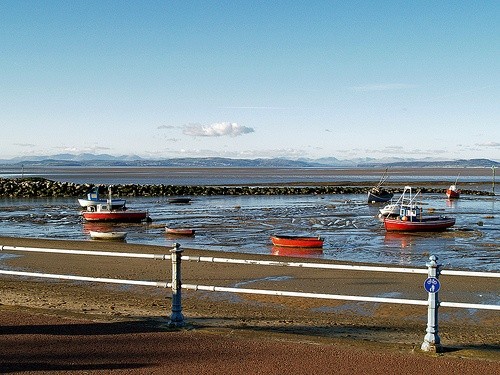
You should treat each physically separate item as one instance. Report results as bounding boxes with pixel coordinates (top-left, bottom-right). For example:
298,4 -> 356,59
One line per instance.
165,227 -> 195,234
166,198 -> 191,203
78,186 -> 126,209
270,235 -> 324,248
379,185 -> 456,232
367,173 -> 394,203
446,173 -> 461,199
89,230 -> 128,240
82,185 -> 149,221
